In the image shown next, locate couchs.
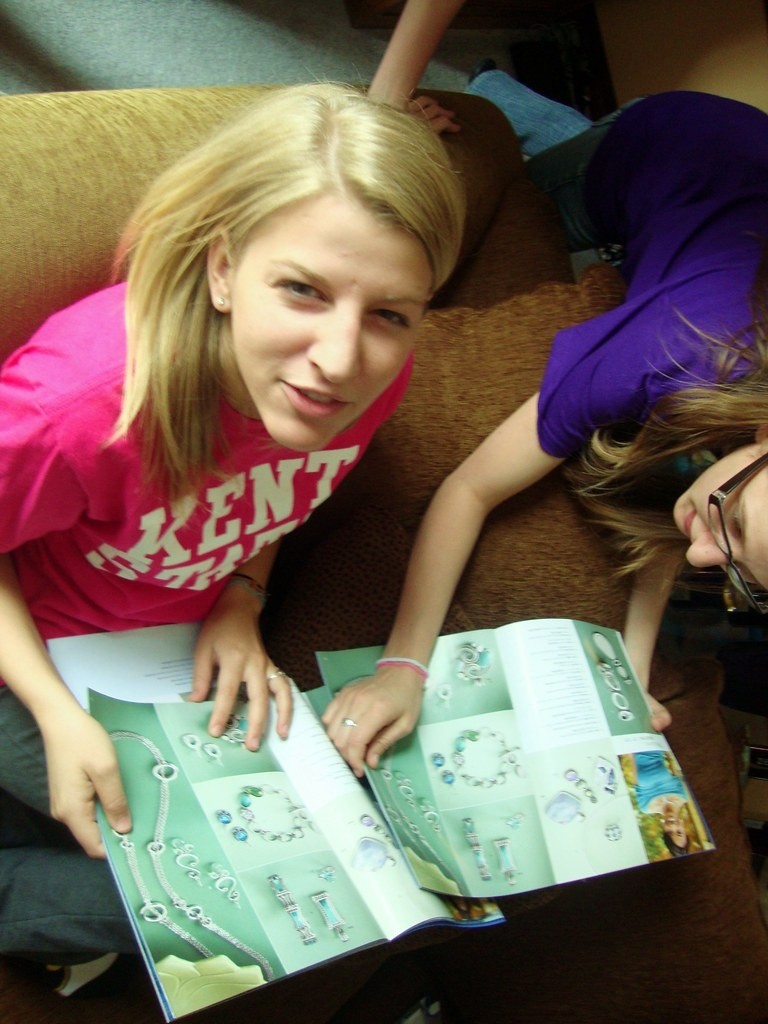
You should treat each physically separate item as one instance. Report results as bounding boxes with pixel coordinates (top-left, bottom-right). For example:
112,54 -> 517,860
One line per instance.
3,86 -> 767,1024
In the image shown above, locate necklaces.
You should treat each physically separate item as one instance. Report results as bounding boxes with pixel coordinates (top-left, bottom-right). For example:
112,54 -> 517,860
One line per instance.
92,731 -> 274,987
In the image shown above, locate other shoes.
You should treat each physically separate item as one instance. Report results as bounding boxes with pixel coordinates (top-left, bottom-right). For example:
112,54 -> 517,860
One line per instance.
43,952 -> 126,1002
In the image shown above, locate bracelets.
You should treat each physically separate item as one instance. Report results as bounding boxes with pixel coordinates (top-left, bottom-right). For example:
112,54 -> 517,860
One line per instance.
376,659 -> 430,680
242,785 -> 313,841
453,727 -> 510,789
231,572 -> 266,606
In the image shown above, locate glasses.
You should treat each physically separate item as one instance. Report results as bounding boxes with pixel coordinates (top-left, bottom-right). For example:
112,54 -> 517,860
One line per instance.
708,452 -> 768,615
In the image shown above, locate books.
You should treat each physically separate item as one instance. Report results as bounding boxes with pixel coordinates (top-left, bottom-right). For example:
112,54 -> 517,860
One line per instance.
77,679 -> 505,1024
316,619 -> 716,896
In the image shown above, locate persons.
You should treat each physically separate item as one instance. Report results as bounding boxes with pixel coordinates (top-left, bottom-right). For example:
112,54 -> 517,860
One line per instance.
622,750 -> 695,858
320,56 -> 768,782
367,0 -> 618,145
0,78 -> 469,861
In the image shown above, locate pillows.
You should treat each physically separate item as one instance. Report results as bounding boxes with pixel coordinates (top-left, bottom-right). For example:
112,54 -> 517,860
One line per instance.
259,266 -> 690,701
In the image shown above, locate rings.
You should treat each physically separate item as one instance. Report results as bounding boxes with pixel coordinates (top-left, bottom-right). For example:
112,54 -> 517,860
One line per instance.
344,720 -> 358,727
267,671 -> 288,681
